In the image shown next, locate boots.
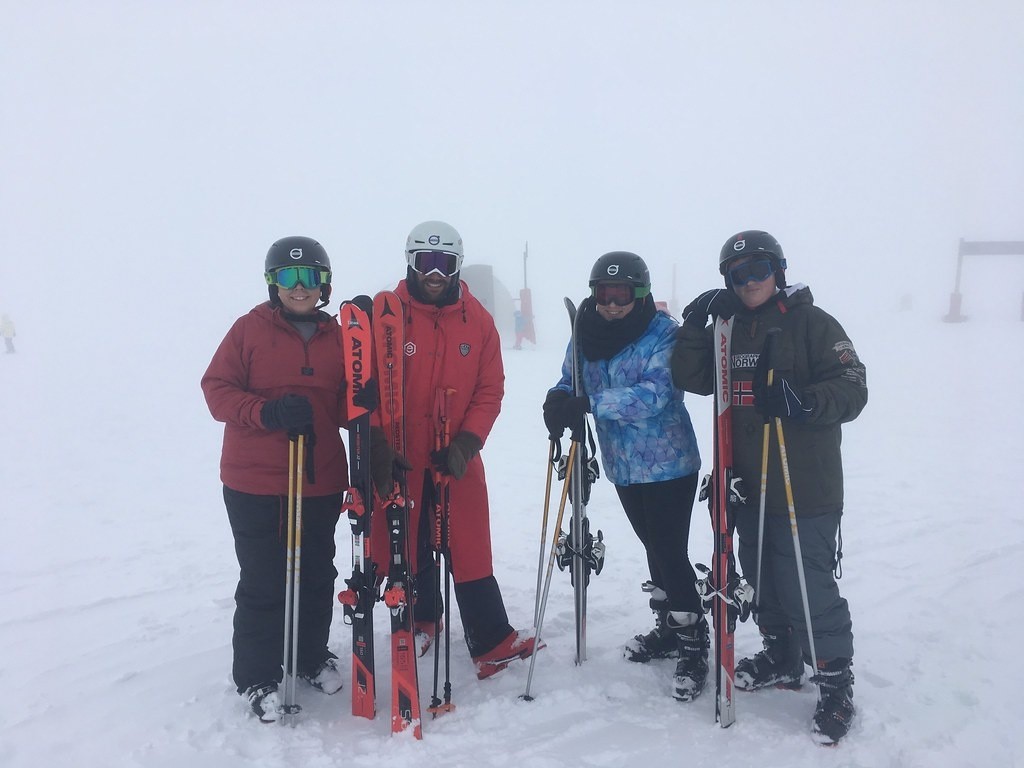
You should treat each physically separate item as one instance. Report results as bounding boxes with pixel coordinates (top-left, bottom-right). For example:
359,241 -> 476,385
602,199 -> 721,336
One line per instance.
666,610 -> 709,702
733,627 -> 803,691
809,657 -> 855,744
624,595 -> 678,662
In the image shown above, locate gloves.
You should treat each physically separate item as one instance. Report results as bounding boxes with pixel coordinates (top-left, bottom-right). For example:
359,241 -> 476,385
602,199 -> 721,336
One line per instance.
681,288 -> 740,328
751,376 -> 813,423
557,395 -> 592,426
362,428 -> 411,499
542,390 -> 571,438
260,393 -> 313,436
431,432 -> 483,480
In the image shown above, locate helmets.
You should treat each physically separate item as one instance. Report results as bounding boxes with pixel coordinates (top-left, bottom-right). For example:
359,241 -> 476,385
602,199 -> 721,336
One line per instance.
264,236 -> 332,308
588,252 -> 650,302
406,221 -> 463,280
719,229 -> 786,294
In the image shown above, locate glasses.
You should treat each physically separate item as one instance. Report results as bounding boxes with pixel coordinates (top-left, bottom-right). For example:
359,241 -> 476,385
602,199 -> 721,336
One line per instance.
729,260 -> 777,287
593,280 -> 634,307
411,248 -> 460,277
269,266 -> 320,290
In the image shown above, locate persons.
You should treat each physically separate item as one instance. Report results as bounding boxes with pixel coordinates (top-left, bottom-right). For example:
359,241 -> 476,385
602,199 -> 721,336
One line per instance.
671,229 -> 867,746
356,221 -> 542,682
201,235 -> 378,726
0,313 -> 17,354
542,251 -> 711,700
513,311 -> 534,350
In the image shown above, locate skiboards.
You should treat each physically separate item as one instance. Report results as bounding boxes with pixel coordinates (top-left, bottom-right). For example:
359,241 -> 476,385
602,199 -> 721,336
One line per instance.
694,312 -> 757,729
334,287 -> 426,747
553,295 -> 608,667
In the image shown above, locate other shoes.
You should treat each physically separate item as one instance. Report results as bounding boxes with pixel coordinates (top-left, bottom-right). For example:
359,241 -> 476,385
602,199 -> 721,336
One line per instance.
293,660 -> 343,694
472,629 -> 548,680
414,617 -> 444,656
245,682 -> 285,723
